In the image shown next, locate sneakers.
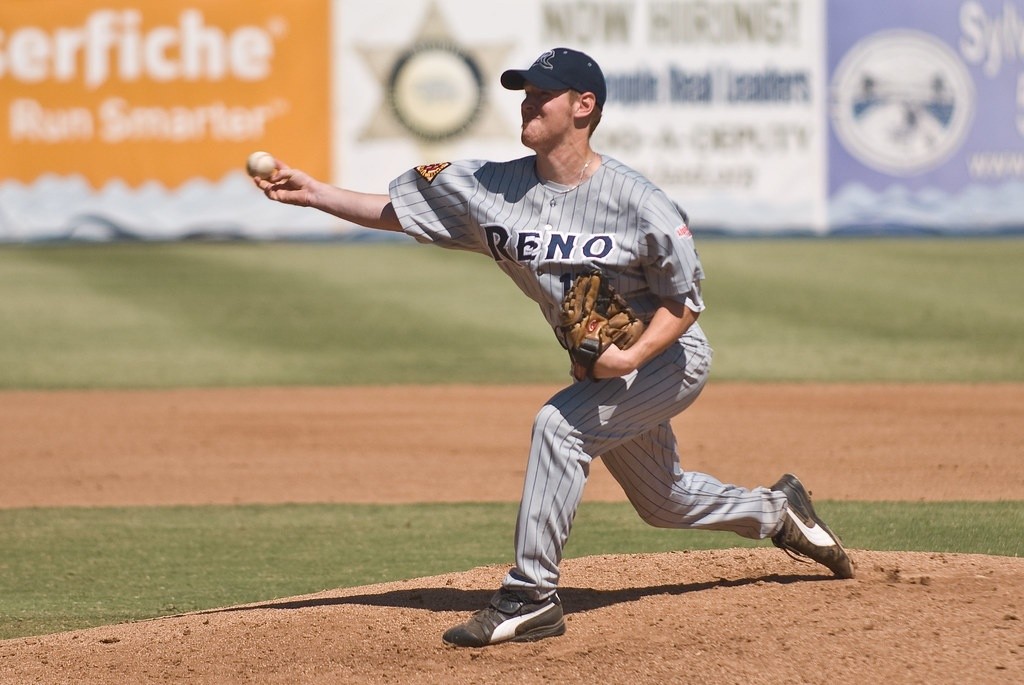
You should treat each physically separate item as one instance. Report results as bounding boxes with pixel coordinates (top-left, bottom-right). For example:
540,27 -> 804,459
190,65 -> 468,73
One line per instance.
441,585 -> 566,647
770,474 -> 856,579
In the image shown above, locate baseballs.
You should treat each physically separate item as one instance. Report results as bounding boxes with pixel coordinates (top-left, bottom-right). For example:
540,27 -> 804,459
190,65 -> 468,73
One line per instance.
243,149 -> 277,179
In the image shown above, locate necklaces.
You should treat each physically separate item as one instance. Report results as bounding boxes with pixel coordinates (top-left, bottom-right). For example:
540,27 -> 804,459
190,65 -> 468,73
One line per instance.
547,152 -> 594,207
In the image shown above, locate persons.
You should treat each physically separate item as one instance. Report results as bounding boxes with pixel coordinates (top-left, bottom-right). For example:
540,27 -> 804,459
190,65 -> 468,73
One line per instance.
251,47 -> 854,647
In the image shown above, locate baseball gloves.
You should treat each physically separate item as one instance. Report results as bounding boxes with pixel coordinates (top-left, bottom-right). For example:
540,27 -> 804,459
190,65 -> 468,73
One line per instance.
554,271 -> 647,382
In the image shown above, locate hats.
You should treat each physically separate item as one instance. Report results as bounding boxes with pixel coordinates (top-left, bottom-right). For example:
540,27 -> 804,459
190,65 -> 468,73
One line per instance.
500,48 -> 607,111
